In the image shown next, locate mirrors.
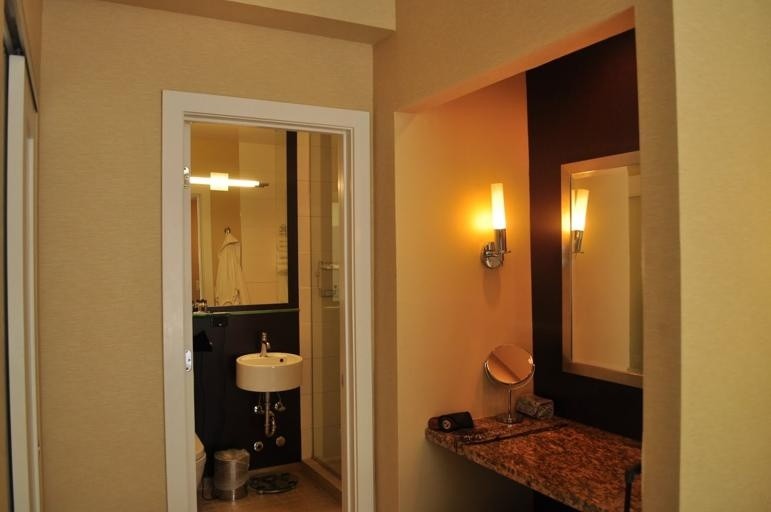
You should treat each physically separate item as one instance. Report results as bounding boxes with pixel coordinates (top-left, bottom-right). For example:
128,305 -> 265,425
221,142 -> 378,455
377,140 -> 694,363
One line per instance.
484,340 -> 538,424
558,149 -> 643,390
185,123 -> 298,312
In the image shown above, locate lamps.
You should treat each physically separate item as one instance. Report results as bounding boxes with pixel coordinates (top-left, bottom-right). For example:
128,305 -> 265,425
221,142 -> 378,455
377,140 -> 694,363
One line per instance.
570,185 -> 590,255
479,178 -> 514,272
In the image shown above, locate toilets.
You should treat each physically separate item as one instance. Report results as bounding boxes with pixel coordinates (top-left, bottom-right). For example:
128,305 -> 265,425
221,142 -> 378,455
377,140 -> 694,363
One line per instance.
195,433 -> 206,488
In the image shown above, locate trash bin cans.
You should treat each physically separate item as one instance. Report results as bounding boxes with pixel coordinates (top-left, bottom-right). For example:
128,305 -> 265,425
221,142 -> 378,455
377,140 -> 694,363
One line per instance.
213,449 -> 250,501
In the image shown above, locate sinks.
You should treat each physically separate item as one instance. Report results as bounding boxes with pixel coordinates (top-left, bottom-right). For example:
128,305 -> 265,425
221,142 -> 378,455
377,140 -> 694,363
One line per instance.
235,353 -> 303,392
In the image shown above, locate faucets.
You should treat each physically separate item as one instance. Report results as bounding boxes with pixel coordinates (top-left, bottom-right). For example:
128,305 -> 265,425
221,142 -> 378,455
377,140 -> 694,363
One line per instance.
259,331 -> 272,357
